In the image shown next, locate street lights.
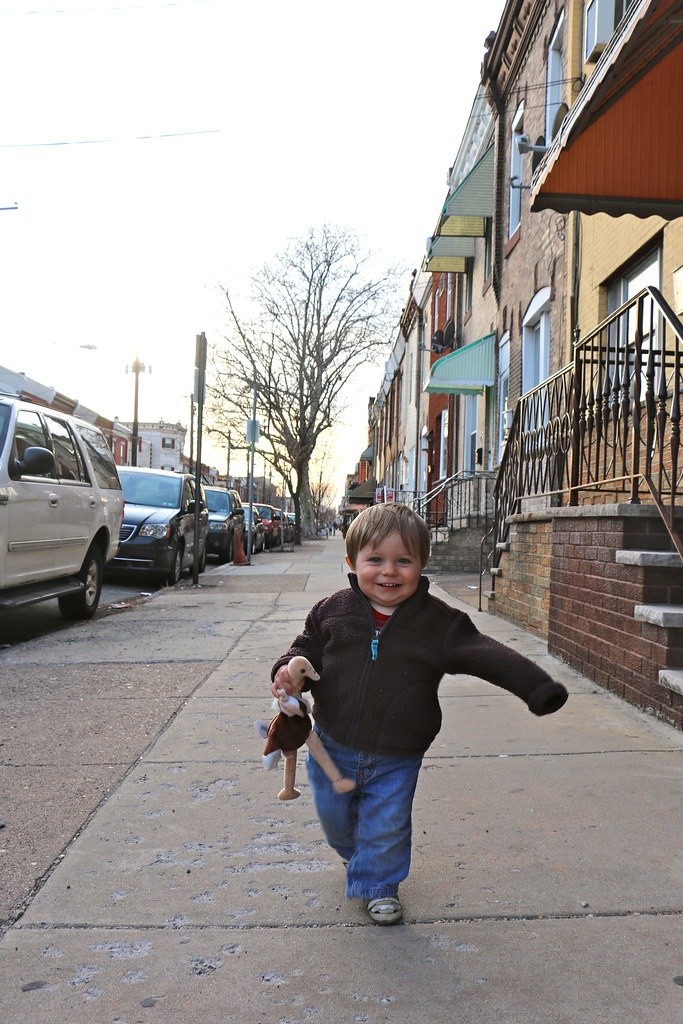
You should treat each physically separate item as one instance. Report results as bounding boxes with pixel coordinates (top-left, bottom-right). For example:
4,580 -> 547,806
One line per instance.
126,357 -> 153,468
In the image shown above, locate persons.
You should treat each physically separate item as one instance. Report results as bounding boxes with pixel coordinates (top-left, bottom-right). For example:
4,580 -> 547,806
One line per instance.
270,502 -> 570,924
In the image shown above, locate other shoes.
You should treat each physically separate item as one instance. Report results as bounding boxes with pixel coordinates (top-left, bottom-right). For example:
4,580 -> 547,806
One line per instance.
364,895 -> 403,923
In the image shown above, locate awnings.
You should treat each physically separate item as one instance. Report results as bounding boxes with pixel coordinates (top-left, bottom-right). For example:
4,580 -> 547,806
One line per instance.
526,0 -> 682,222
434,143 -> 493,238
424,332 -> 496,395
348,477 -> 376,503
421,238 -> 477,274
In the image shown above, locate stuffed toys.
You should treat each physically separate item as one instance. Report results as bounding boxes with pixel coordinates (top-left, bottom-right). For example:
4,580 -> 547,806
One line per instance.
254,656 -> 356,802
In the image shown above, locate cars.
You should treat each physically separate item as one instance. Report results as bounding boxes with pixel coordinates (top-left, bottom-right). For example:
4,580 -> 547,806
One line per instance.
240,505 -> 266,555
274,508 -> 296,545
241,502 -> 281,549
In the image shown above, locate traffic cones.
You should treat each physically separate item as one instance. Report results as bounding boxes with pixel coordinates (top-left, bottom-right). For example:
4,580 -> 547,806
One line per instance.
229,528 -> 252,567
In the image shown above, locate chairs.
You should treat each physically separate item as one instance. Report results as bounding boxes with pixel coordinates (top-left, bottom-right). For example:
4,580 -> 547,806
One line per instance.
215,496 -> 227,510
145,479 -> 177,506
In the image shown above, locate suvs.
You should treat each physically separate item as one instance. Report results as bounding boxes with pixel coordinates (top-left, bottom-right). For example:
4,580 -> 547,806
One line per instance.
201,484 -> 247,565
105,463 -> 210,587
0,395 -> 125,620
